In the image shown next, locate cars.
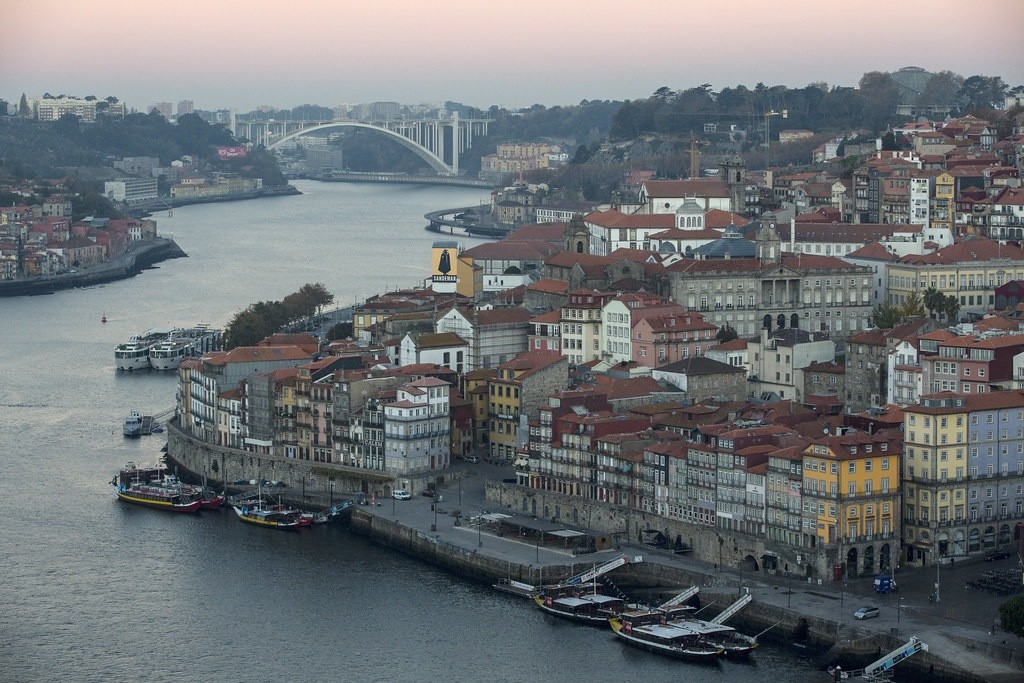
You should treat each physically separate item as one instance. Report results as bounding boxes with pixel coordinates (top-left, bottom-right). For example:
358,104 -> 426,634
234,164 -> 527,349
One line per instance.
853,606 -> 881,621
983,551 -> 1010,562
422,488 -> 446,503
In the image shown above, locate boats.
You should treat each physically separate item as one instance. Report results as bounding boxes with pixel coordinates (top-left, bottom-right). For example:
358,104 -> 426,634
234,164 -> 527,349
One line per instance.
148,320 -> 224,372
108,458 -> 331,532
835,664 -> 897,683
123,408 -> 144,437
827,665 -> 849,680
114,327 -> 184,371
490,560 -> 760,665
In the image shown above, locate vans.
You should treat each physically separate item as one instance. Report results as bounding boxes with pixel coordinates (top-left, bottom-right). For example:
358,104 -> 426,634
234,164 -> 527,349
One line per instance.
391,490 -> 411,501
463,455 -> 480,465
872,575 -> 898,595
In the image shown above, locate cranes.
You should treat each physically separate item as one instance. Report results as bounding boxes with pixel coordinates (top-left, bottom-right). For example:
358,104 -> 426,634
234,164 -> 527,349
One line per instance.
640,129 -> 707,179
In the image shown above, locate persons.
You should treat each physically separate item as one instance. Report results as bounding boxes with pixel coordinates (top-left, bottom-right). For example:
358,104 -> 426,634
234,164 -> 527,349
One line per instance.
951,557 -> 954,566
783,564 -> 787,576
